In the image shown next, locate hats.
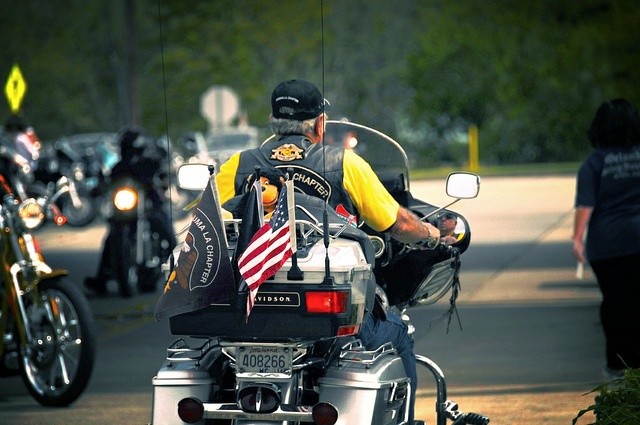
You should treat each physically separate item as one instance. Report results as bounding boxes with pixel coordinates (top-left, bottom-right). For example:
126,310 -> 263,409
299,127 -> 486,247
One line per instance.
271,80 -> 323,121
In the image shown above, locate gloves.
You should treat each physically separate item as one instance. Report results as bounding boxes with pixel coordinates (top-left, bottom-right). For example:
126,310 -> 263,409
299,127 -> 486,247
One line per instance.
409,222 -> 441,251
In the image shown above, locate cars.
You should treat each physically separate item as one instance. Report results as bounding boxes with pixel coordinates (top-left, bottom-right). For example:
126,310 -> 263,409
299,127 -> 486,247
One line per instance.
194,128 -> 258,167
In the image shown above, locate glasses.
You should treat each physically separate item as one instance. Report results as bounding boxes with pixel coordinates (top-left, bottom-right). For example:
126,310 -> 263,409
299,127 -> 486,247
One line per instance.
445,213 -> 455,220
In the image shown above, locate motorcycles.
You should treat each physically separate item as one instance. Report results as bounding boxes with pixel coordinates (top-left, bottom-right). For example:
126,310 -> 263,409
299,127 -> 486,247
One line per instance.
152,121 -> 489,424
91,144 -> 176,297
26,142 -> 99,226
1,192 -> 97,407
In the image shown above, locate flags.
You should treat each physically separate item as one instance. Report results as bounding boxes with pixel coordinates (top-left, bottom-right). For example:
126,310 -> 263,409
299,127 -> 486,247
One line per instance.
237,178 -> 300,327
230,178 -> 265,328
154,172 -> 238,324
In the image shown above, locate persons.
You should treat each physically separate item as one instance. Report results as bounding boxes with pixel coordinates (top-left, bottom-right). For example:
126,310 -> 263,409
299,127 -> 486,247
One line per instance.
84,131 -> 173,296
439,213 -> 465,245
210,79 -> 442,425
572,98 -> 640,379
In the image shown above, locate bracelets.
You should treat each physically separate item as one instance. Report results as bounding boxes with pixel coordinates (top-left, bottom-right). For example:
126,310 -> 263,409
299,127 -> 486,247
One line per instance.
421,221 -> 432,243
